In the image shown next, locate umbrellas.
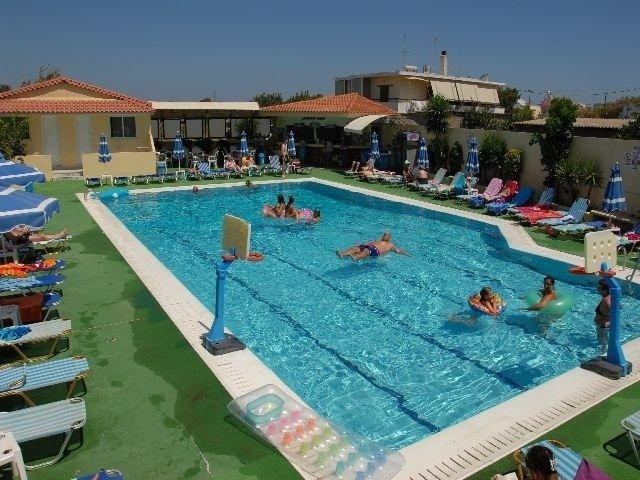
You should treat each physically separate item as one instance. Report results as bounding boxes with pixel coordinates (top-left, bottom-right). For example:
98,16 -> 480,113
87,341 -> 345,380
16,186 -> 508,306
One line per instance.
602,160 -> 628,226
0,159 -> 46,193
415,137 -> 431,170
464,138 -> 481,176
173,129 -> 185,171
287,129 -> 298,159
239,129 -> 250,157
0,183 -> 62,265
98,131 -> 111,185
368,130 -> 382,161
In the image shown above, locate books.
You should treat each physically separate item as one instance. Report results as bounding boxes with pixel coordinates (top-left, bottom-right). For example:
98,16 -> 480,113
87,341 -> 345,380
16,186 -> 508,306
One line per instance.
489,472 -> 520,479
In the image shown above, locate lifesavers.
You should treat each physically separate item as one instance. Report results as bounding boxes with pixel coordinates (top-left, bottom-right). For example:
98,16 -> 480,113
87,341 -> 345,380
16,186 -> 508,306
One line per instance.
469,294 -> 505,315
263,205 -> 313,219
528,290 -> 573,314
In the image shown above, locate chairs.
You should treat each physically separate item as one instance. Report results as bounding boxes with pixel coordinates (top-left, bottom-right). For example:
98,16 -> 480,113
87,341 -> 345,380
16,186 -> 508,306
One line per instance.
85,146 -> 302,186
514,440 -> 612,480
620,410 -> 640,462
345,168 -> 640,252
0,234 -> 90,480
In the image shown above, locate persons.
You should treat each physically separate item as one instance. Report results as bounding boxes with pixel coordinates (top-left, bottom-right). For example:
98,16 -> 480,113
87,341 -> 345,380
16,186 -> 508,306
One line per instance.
447,286 -> 502,325
322,136 -> 335,166
349,160 -> 371,173
481,178 -> 511,203
335,231 -> 410,262
402,160 -> 414,188
413,165 -> 429,184
519,275 -> 557,335
592,278 -> 624,352
240,153 -> 259,173
157,153 -> 168,183
3,223 -> 68,242
525,444 -> 559,480
263,194 -> 287,219
285,195 -> 301,222
225,154 -> 244,174
279,138 -> 289,177
299,137 -> 306,160
305,209 -> 320,223
